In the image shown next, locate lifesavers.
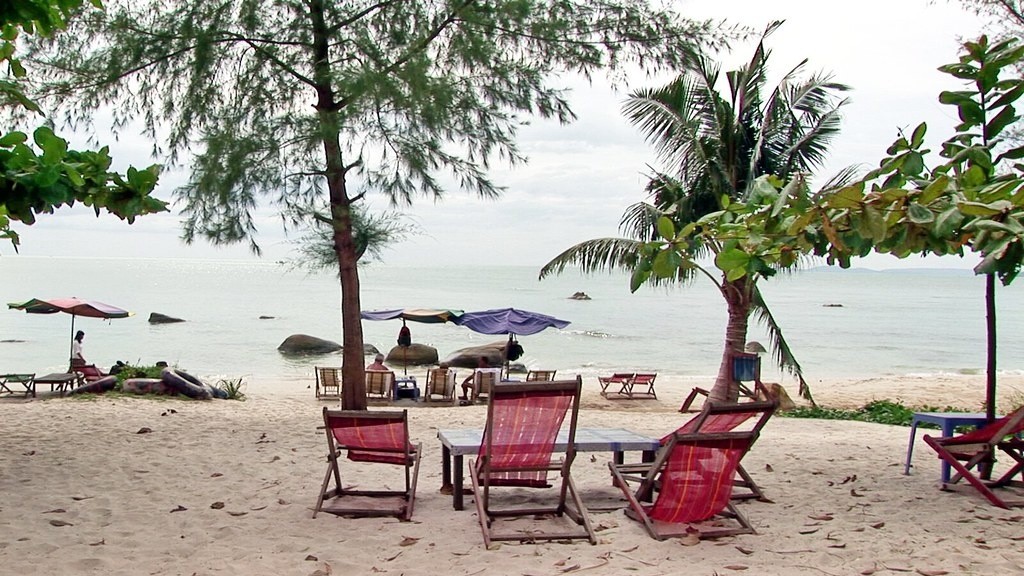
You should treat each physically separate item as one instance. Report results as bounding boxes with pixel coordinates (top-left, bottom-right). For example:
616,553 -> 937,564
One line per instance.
67,375 -> 119,397
161,367 -> 212,399
124,378 -> 179,396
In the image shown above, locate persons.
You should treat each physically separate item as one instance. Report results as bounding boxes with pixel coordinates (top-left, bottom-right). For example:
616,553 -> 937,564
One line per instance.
428,362 -> 456,402
458,356 -> 493,400
366,354 -> 389,370
72,330 -> 86,366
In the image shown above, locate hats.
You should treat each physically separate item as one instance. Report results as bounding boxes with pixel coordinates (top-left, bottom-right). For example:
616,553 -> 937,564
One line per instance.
440,362 -> 448,367
375,354 -> 384,360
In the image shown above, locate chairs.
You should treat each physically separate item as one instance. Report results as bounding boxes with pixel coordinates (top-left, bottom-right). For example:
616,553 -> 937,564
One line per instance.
315,365 -> 556,403
0,373 -> 35,399
73,364 -> 107,384
313,407 -> 422,522
599,367 -> 781,542
469,375 -> 597,550
923,405 -> 1024,510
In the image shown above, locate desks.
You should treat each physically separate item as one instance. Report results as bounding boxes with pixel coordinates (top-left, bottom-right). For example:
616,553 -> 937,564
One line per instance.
438,428 -> 660,511
393,380 -> 417,400
906,413 -> 1024,482
33,373 -> 82,397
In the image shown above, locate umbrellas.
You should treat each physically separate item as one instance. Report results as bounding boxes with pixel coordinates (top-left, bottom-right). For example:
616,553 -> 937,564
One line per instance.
453,307 -> 572,380
361,307 -> 464,387
7,296 -> 136,375
